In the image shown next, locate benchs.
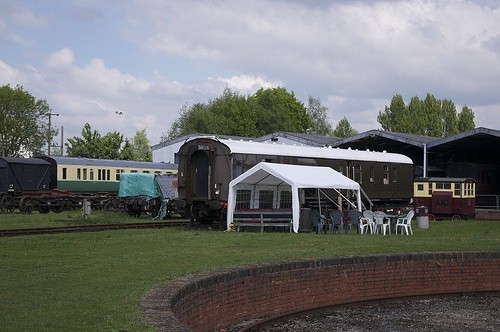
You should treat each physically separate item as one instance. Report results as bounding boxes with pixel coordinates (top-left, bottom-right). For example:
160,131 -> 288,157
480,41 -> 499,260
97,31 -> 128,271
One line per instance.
231,209 -> 292,233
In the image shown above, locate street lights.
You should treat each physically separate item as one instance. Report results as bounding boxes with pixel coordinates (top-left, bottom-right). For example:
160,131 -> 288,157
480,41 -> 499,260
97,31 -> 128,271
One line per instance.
38,113 -> 60,155
114,110 -> 122,156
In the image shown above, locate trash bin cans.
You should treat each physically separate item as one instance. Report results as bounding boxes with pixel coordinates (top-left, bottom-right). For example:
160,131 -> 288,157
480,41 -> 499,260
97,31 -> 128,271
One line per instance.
416,206 -> 429,229
298,208 -> 312,233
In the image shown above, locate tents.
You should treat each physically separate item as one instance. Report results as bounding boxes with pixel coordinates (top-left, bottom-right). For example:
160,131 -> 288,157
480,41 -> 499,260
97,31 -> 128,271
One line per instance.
226,162 -> 363,234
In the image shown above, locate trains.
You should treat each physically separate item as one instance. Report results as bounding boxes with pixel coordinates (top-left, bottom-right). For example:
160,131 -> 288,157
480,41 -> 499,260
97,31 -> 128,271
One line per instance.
413,176 -> 477,223
177,137 -> 418,235
1,156 -> 176,215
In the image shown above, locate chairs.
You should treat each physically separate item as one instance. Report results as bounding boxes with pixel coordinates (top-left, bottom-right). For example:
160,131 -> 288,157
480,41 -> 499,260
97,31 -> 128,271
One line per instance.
311,209 -> 414,235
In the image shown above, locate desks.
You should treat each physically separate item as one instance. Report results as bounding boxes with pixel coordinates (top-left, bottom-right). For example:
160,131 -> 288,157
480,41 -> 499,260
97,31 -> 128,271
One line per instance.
385,214 -> 398,232
